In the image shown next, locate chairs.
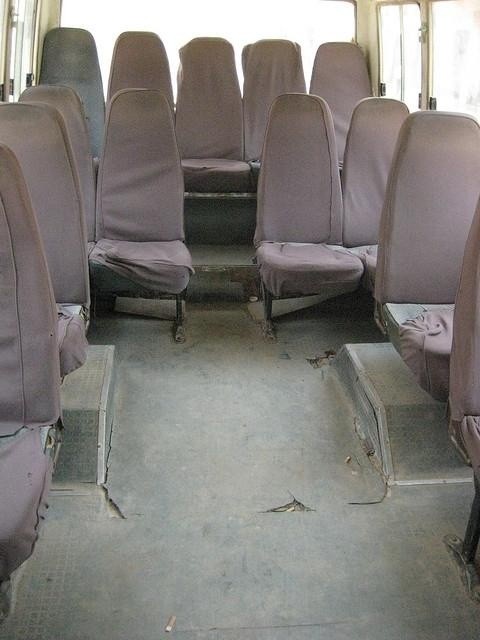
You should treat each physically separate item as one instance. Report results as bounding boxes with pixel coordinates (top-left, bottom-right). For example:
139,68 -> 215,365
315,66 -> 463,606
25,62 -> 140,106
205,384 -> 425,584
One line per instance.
0,144 -> 65,631
374,110 -> 480,463
442,195 -> 480,602
337,92 -> 411,289
104,34 -> 174,118
175,36 -> 252,203
306,41 -> 374,176
249,90 -> 366,343
86,86 -> 197,341
38,26 -> 102,169
0,98 -> 90,382
18,85 -> 96,270
236,39 -> 309,198
